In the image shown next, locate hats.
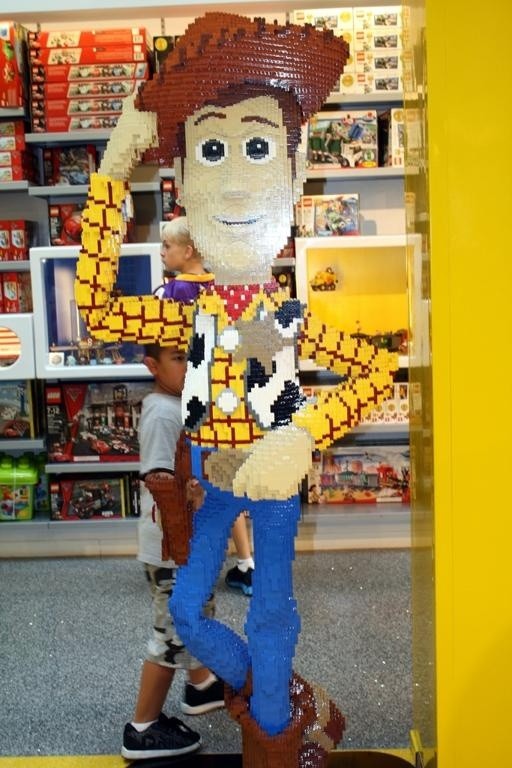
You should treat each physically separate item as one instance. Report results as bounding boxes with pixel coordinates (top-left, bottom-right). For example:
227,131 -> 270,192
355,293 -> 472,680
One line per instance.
135,4 -> 353,165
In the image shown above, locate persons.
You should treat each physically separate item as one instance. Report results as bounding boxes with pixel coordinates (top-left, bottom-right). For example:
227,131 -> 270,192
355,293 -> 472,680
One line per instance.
157,213 -> 255,597
119,339 -> 228,763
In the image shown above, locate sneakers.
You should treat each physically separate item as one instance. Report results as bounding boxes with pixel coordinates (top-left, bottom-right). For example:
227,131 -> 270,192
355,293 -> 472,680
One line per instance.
119,711 -> 204,762
178,678 -> 227,716
225,564 -> 254,598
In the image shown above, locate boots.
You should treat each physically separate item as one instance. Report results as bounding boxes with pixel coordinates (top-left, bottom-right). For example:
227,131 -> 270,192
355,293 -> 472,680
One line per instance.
214,657 -> 367,753
